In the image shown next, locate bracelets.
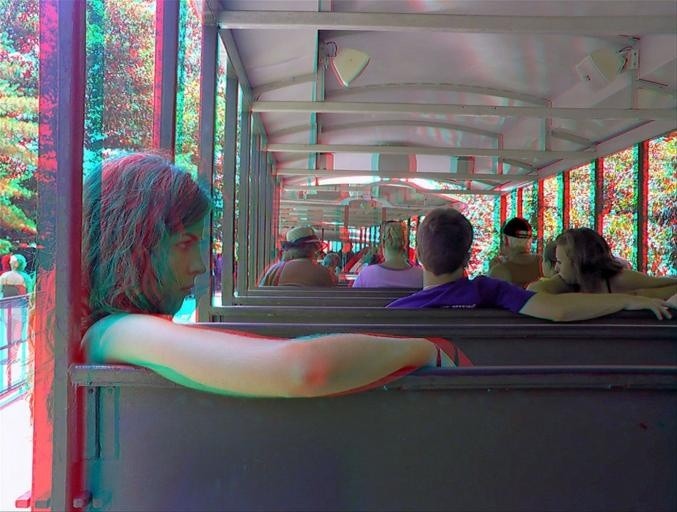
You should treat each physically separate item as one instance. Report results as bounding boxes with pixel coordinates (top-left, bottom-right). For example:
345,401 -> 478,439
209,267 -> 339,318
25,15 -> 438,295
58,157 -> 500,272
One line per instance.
434,344 -> 443,368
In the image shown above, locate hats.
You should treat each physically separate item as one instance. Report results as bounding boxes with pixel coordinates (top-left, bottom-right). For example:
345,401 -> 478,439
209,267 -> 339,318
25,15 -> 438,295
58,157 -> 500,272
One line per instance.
280,227 -> 327,252
503,218 -> 532,239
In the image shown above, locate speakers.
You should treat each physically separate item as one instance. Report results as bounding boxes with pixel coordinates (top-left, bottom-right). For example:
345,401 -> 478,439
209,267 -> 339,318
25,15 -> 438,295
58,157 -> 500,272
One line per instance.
575,48 -> 626,91
316,153 -> 334,170
333,48 -> 370,87
457,159 -> 476,175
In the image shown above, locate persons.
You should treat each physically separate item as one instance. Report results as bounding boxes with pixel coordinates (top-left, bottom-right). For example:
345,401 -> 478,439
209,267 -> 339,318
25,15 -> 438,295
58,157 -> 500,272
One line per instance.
214,254 -> 222,291
487,216 -> 543,289
384,205 -> 677,322
351,220 -> 423,288
314,239 -> 355,275
525,240 -> 558,292
528,226 -> 677,301
27,150 -> 472,400
0,252 -> 34,366
257,225 -> 339,287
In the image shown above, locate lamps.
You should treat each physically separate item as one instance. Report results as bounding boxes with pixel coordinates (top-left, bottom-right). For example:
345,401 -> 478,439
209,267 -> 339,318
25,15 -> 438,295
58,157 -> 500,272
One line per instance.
573,45 -> 635,94
318,41 -> 371,89
